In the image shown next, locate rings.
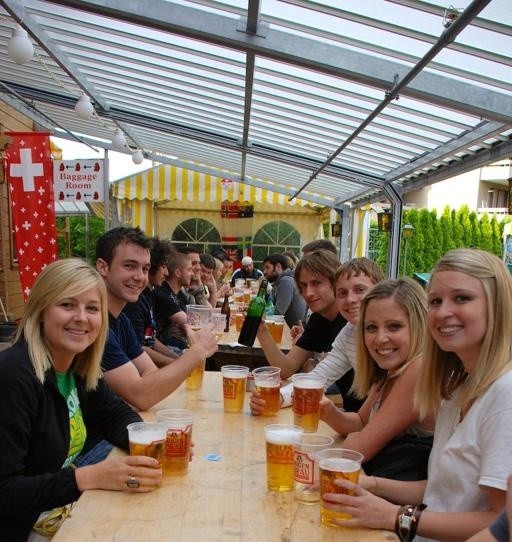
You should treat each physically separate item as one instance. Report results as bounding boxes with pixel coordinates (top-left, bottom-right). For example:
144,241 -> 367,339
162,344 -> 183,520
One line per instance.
127,476 -> 140,488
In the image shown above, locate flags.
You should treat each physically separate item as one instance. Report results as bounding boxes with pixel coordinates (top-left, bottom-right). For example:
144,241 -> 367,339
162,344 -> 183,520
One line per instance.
221,205 -> 238,218
239,205 -> 254,217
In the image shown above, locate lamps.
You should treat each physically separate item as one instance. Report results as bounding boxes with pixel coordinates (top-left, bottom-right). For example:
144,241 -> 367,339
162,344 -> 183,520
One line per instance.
113,129 -> 124,146
132,149 -> 143,164
75,95 -> 94,119
8,30 -> 33,64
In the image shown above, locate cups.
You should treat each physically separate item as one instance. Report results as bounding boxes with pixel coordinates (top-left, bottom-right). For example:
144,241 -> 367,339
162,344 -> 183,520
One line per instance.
227,278 -> 286,344
291,373 -> 327,433
212,314 -> 226,339
185,304 -> 214,348
157,409 -> 193,481
182,349 -> 206,389
125,422 -> 167,491
313,448 -> 365,529
295,432 -> 333,505
263,423 -> 304,492
221,365 -> 249,413
252,366 -> 283,417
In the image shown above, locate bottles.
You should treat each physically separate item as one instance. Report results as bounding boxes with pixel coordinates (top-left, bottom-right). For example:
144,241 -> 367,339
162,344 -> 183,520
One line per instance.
236,279 -> 268,347
220,294 -> 230,332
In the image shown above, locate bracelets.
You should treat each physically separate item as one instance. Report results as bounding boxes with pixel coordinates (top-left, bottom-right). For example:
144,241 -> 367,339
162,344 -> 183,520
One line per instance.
395,504 -> 428,542
489,510 -> 512,542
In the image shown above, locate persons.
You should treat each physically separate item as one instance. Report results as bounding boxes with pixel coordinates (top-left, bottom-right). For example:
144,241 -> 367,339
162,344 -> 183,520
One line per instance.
464,476 -> 512,542
322,249 -> 512,542
121,238 -> 236,356
230,256 -> 263,287
0,258 -> 195,542
290,278 -> 439,481
249,239 -> 385,416
72,227 -> 219,467
